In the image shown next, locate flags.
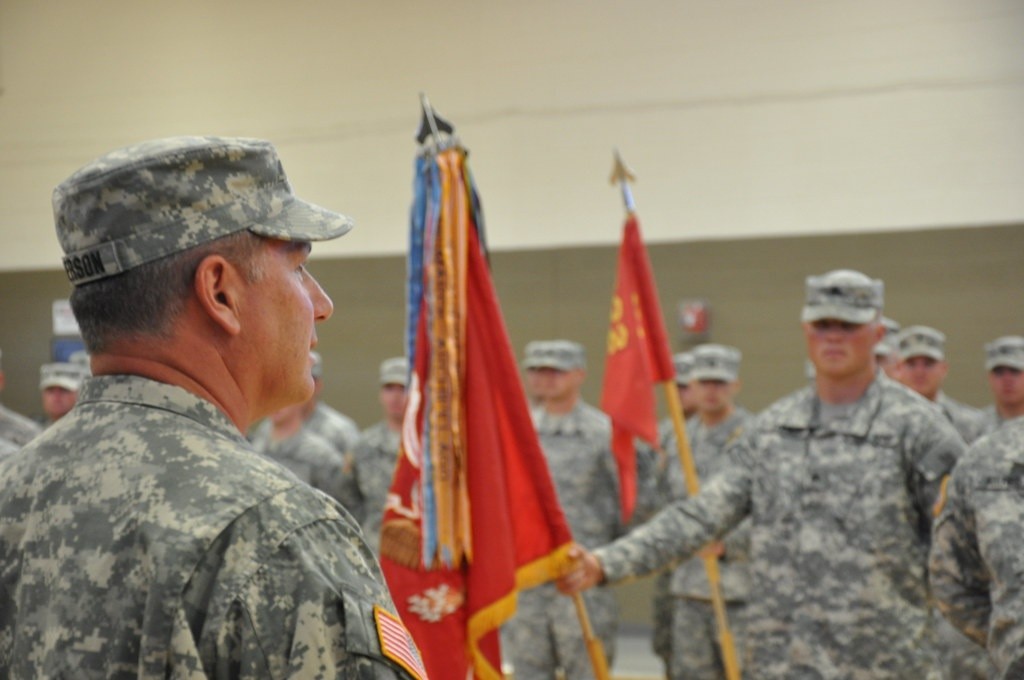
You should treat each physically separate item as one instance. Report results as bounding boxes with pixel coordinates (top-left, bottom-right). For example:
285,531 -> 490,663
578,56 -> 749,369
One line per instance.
602,218 -> 676,522
378,138 -> 577,680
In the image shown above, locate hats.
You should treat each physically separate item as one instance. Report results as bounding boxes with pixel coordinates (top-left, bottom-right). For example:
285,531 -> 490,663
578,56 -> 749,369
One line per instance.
51,136 -> 352,285
307,351 -> 324,379
689,343 -> 743,383
378,356 -> 410,386
38,362 -> 85,392
670,350 -> 695,385
799,268 -> 883,324
984,336 -> 1024,370
875,316 -> 901,354
518,340 -> 585,374
895,327 -> 947,362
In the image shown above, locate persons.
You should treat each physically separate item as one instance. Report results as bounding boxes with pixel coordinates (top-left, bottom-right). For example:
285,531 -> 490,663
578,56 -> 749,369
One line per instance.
500,340 -> 621,680
258,352 -> 355,495
337,357 -> 410,551
979,335 -> 1024,421
0,136 -> 427,680
558,267 -> 968,680
39,362 -> 86,425
635,342 -> 753,680
927,419 -> 1024,680
876,316 -> 998,446
